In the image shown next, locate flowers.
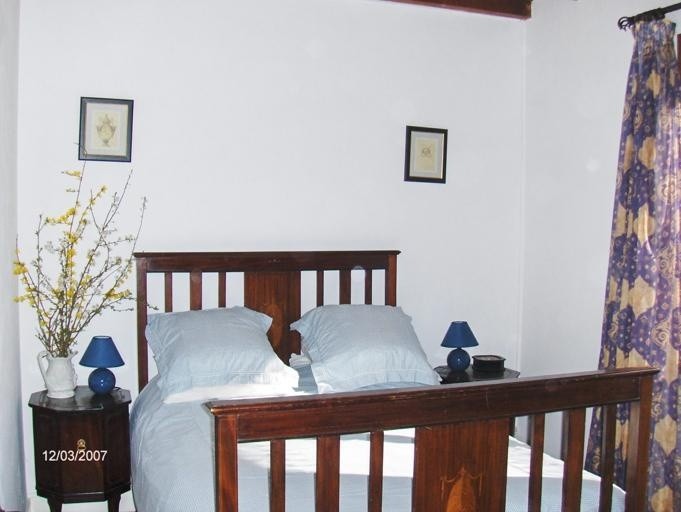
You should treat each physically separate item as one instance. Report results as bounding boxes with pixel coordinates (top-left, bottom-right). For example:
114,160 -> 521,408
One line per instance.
12,144 -> 148,357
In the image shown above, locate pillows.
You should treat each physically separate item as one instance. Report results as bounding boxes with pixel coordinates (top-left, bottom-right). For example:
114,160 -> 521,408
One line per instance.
290,305 -> 441,393
143,307 -> 300,402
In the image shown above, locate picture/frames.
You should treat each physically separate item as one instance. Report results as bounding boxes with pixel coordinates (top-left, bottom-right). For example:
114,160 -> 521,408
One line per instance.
78,97 -> 134,163
404,125 -> 448,184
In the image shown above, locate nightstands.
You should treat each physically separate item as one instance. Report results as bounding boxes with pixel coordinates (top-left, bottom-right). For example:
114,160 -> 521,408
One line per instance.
28,384 -> 132,511
434,364 -> 521,438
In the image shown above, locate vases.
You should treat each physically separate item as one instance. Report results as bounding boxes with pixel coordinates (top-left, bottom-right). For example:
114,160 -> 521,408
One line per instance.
38,350 -> 82,399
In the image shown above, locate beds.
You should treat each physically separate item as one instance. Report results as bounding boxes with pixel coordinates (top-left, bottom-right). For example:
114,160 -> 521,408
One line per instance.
131,250 -> 661,512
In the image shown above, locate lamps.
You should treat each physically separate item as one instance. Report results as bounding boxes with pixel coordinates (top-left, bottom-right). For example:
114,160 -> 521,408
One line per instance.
79,335 -> 125,394
441,320 -> 480,371
